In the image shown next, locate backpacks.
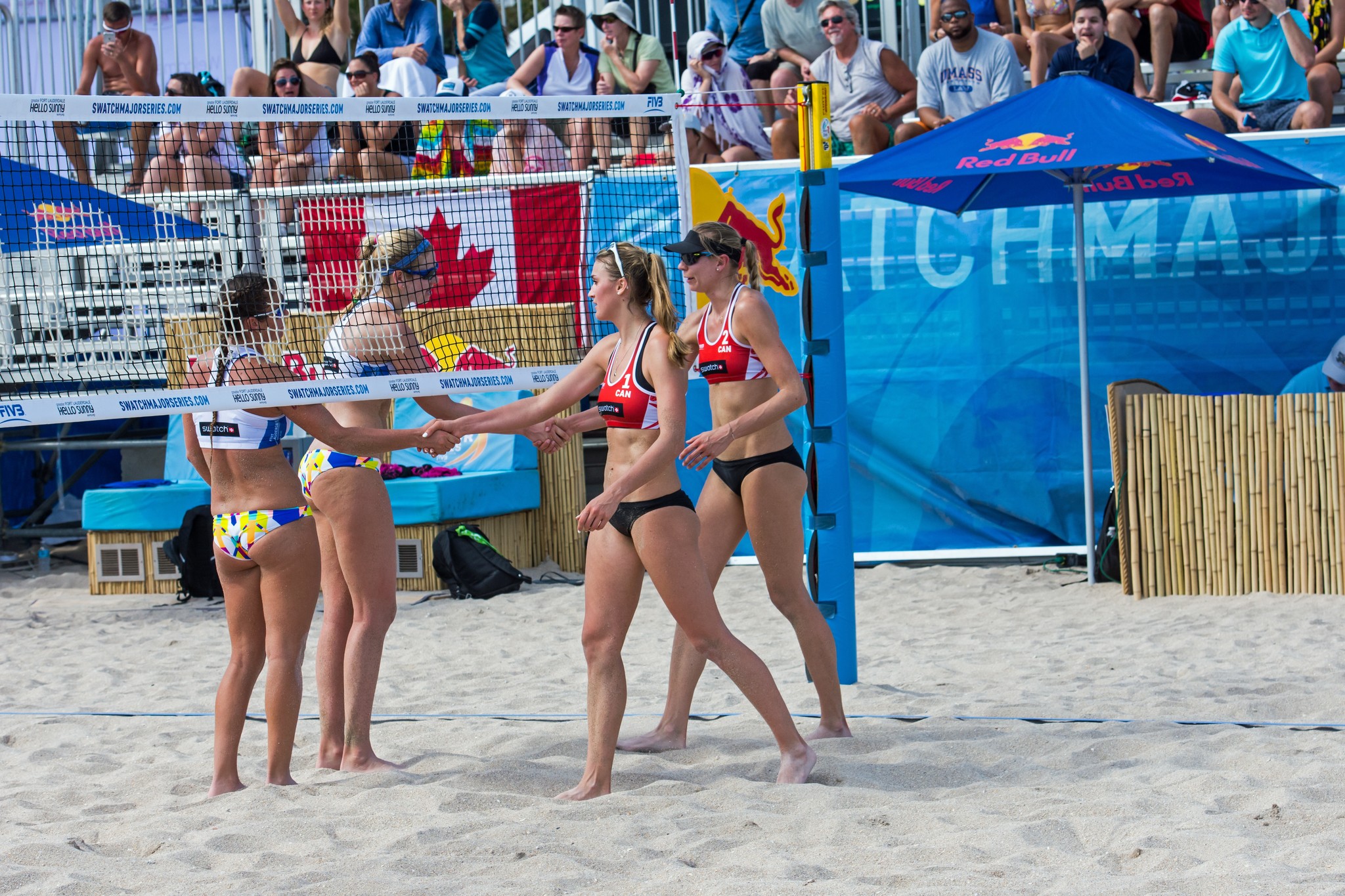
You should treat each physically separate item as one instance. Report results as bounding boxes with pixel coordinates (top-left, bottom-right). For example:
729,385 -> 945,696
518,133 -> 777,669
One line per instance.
162,504 -> 223,598
1092,487 -> 1121,583
431,524 -> 532,601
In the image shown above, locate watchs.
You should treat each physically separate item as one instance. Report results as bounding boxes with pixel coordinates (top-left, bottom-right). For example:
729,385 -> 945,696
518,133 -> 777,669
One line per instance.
452,10 -> 463,18
933,30 -> 940,41
769,51 -> 778,60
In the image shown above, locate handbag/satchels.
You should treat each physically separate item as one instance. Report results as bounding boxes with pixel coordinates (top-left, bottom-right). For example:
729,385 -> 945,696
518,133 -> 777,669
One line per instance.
196,70 -> 225,97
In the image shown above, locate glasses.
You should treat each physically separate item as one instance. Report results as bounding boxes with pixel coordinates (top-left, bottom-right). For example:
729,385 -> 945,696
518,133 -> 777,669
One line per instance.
941,10 -> 968,23
553,25 -> 581,32
605,242 -> 629,290
701,49 -> 722,61
274,77 -> 301,87
1241,0 -> 1260,4
103,20 -> 131,35
598,16 -> 616,25
681,252 -> 714,266
347,70 -> 373,80
382,262 -> 439,280
820,15 -> 849,27
247,293 -> 285,319
165,87 -> 184,97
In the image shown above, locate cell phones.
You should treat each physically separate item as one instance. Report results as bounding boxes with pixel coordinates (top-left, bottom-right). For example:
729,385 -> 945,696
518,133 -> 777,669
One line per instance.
103,32 -> 116,45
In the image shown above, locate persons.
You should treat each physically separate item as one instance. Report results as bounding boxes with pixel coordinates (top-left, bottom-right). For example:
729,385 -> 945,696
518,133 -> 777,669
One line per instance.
179,220 -> 855,803
54,0 -> 1345,226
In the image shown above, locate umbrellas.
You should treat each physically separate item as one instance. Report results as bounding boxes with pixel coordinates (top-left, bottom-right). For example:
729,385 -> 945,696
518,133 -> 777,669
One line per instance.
834,72 -> 1341,583
1,157 -> 234,503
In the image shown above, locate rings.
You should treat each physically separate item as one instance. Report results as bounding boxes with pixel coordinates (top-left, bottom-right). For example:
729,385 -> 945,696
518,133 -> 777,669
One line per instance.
872,109 -> 877,113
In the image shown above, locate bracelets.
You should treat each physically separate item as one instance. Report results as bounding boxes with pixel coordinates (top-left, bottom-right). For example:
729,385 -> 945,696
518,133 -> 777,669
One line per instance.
1276,10 -> 1290,19
283,121 -> 292,123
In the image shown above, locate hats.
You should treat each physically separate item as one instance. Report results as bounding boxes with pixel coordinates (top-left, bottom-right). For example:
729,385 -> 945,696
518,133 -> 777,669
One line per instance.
1321,335 -> 1345,386
701,42 -> 726,53
590,1 -> 642,35
434,77 -> 469,97
499,88 -> 525,97
662,230 -> 741,262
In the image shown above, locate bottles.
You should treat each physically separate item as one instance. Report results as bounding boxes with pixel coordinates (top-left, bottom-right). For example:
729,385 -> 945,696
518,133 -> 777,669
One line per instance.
1106,526 -> 1118,540
38,541 -> 50,571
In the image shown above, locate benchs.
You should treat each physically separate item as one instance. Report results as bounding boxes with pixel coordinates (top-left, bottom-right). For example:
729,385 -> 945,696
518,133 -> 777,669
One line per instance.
81,389 -> 540,594
1024,50 -> 1345,116
0,233 -> 312,382
